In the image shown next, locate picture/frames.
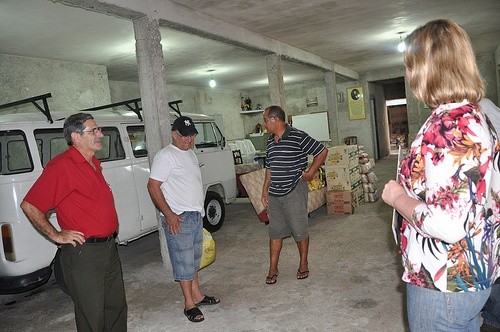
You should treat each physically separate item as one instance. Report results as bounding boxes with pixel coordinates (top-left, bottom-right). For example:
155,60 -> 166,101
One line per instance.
346,86 -> 366,120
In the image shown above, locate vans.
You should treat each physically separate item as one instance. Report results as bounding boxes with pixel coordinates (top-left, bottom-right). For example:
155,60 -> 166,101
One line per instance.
0,111 -> 237,297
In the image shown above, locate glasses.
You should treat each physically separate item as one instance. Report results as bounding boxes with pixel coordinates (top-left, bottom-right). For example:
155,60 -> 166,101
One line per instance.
76,127 -> 102,133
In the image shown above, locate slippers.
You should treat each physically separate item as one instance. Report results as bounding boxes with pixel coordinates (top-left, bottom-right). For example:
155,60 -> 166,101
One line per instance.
266,274 -> 278,284
195,295 -> 221,305
184,305 -> 204,322
296,269 -> 309,279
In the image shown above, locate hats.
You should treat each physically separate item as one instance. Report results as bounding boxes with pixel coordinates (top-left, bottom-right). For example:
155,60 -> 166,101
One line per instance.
171,116 -> 199,136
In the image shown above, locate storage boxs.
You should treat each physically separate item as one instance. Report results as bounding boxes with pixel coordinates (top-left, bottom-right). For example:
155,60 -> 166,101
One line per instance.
326,145 -> 365,216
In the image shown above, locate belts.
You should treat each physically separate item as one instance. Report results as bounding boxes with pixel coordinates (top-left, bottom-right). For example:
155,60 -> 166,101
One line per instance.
82,231 -> 118,243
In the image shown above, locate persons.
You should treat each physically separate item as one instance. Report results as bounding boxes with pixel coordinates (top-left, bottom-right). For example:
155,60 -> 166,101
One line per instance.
19,111 -> 129,332
381,18 -> 500,332
147,116 -> 221,322
260,104 -> 329,285
